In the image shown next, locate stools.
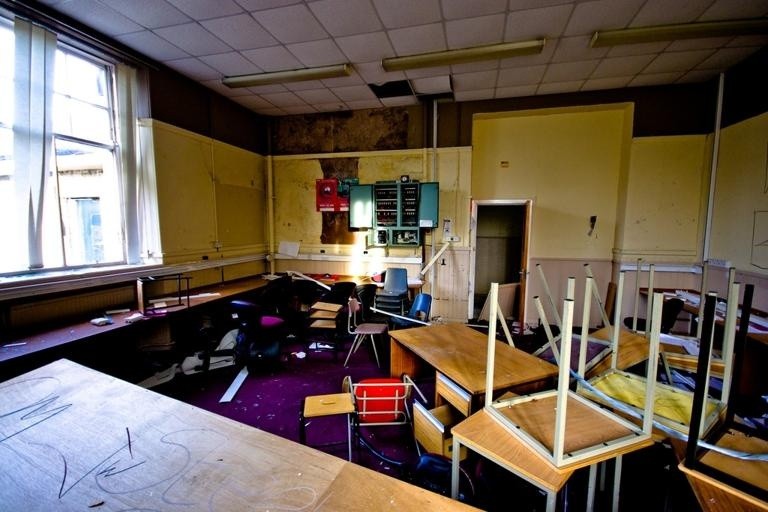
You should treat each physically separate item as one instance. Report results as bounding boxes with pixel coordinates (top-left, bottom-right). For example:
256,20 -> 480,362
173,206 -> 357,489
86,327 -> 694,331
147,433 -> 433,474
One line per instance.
134,272 -> 194,315
293,391 -> 362,465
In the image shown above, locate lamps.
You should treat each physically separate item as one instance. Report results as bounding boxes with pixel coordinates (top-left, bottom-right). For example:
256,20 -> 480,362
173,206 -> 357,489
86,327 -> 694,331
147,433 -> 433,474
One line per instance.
591,18 -> 768,46
382,37 -> 545,71
221,65 -> 352,90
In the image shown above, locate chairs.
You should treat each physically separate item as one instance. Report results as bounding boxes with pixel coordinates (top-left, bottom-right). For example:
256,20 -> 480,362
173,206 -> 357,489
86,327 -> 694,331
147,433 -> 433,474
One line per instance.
408,452 -> 476,506
227,266 -> 433,371
338,371 -> 427,462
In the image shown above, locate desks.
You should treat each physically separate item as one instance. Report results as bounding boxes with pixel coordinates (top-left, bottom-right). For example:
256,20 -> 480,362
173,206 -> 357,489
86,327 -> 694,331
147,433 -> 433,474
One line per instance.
0,356 -> 481,512
386,318 -> 561,462
451,258 -> 768,510
1,270 -> 410,372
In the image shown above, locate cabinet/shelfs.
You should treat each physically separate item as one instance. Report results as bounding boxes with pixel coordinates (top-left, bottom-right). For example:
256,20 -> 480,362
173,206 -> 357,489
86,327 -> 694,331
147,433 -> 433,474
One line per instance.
348,180 -> 441,228
374,229 -> 421,247
